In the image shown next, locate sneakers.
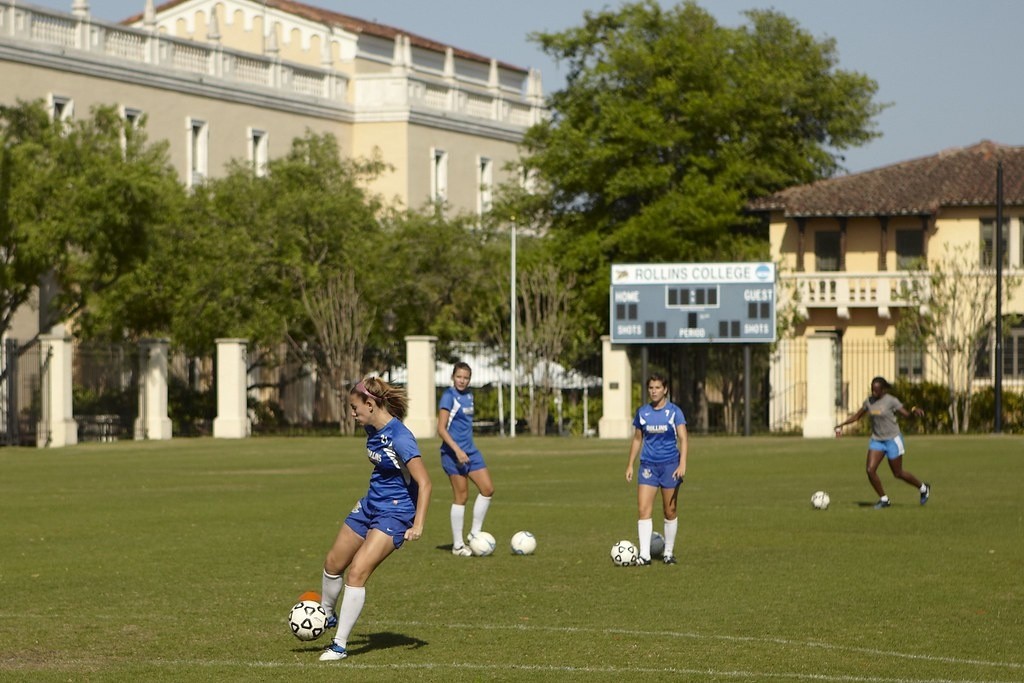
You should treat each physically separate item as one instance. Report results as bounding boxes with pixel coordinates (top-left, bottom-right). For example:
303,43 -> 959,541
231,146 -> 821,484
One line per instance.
467,533 -> 475,542
632,556 -> 652,566
318,637 -> 347,661
663,555 -> 677,564
920,483 -> 931,505
452,546 -> 473,557
324,612 -> 338,628
873,499 -> 891,511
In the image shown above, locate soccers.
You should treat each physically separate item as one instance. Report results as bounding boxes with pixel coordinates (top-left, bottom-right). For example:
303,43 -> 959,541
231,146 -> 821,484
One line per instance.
510,531 -> 537,555
610,539 -> 639,567
810,490 -> 830,510
288,599 -> 329,641
470,532 -> 496,556
650,531 -> 665,555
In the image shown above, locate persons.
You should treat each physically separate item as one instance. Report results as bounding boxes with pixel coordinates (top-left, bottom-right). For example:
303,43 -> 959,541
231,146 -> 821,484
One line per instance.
438,364 -> 495,556
834,377 -> 930,507
625,373 -> 688,567
320,378 -> 433,662
